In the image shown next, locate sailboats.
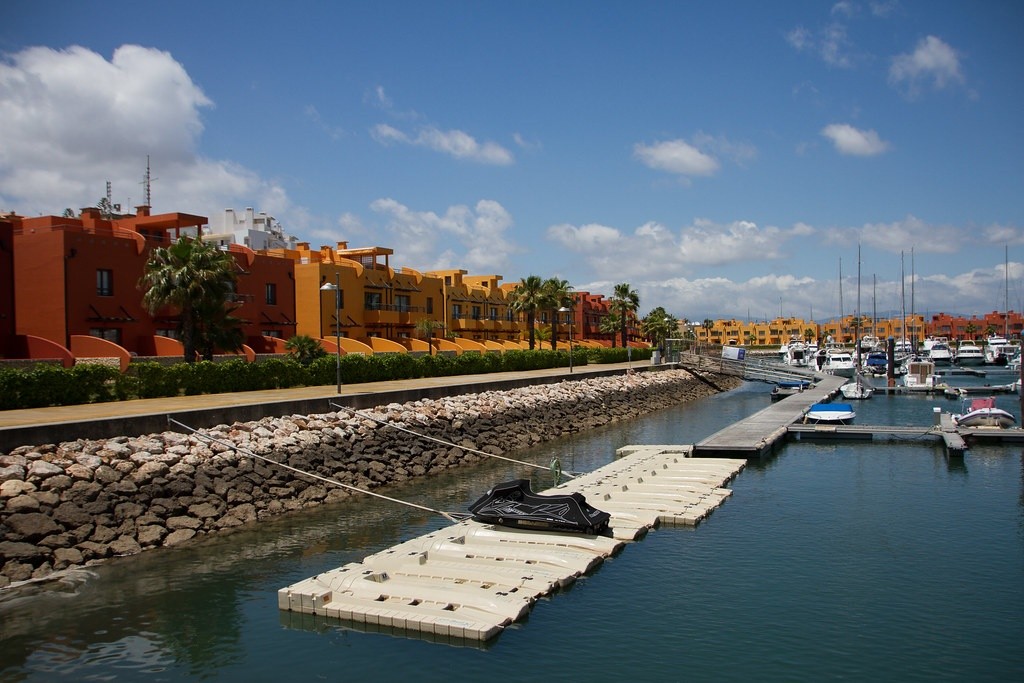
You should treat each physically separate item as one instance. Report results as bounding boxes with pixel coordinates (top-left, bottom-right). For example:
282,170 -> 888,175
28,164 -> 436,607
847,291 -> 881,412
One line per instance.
777,242 -> 1024,390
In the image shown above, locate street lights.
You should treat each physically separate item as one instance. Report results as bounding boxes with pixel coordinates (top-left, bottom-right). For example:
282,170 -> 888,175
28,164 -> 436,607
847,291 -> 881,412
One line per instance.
558,299 -> 573,373
318,272 -> 344,394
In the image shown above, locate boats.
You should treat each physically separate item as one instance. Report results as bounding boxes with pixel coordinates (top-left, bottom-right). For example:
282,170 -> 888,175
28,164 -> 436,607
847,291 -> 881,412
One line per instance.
804,402 -> 857,424
956,396 -> 1017,427
769,380 -> 811,400
